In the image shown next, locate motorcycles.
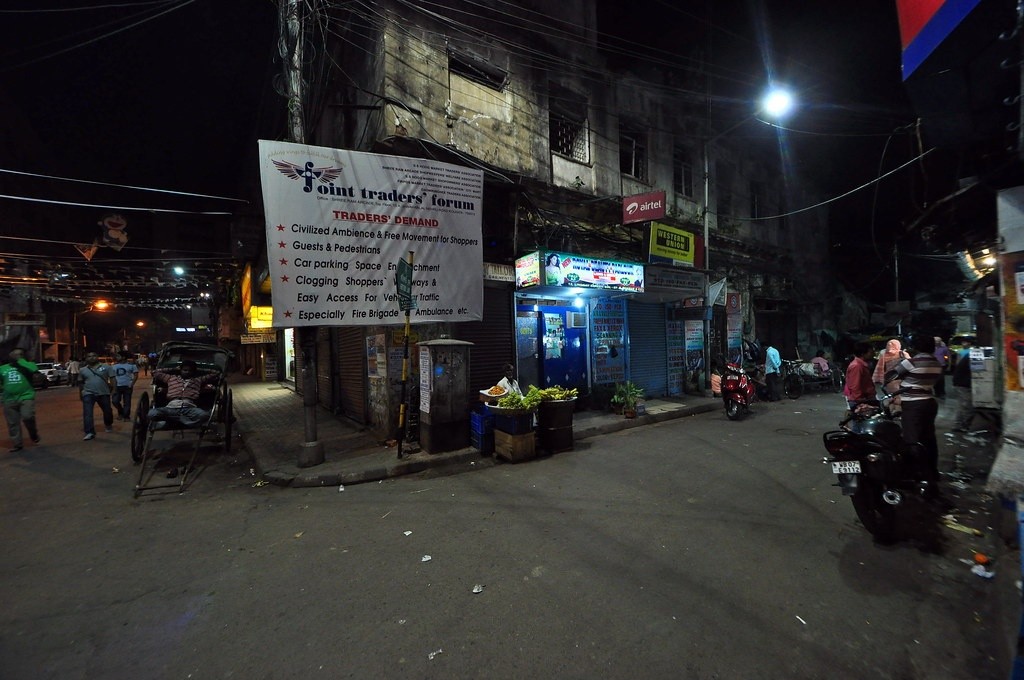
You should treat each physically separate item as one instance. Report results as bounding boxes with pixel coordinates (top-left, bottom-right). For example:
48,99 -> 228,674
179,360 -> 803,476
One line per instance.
820,369 -> 931,540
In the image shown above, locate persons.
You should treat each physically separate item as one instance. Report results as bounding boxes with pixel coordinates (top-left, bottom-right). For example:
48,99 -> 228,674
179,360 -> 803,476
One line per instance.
60,356 -> 80,387
144,355 -> 160,376
0,348 -> 41,452
760,341 -> 783,402
112,351 -> 139,422
77,350 -> 116,440
842,336 -> 912,433
810,350 -> 844,376
496,364 -> 537,426
933,335 -> 972,396
147,360 -> 221,430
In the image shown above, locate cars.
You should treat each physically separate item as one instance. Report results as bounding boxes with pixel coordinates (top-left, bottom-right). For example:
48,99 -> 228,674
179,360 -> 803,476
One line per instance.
127,353 -> 148,366
53,364 -> 72,385
32,371 -> 49,389
98,357 -> 118,365
870,336 -> 910,365
36,363 -> 62,385
948,331 -> 977,359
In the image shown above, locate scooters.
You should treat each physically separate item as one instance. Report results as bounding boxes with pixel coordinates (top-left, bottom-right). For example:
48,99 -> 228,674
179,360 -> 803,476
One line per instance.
716,355 -> 757,420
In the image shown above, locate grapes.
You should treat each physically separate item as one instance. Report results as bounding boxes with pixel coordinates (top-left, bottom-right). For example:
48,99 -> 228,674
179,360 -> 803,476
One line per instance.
499,385 -> 542,409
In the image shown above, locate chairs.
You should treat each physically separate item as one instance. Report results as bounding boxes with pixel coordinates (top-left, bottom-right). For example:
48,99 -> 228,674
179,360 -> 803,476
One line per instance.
157,362 -> 223,429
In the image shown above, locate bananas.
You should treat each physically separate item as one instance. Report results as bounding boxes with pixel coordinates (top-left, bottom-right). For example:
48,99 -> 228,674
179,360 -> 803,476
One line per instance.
539,387 -> 579,400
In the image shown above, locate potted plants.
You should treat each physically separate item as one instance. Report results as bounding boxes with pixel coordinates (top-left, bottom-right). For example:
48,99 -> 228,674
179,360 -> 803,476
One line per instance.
609,381 -> 646,418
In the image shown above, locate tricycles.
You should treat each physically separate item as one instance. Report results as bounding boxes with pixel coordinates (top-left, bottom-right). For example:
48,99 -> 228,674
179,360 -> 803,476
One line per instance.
782,359 -> 842,400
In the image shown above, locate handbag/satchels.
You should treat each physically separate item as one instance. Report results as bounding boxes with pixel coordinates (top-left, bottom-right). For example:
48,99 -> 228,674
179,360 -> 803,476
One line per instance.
107,383 -> 113,393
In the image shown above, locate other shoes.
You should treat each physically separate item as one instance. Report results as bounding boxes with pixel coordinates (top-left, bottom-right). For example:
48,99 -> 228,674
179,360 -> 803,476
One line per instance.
83,433 -> 95,440
166,467 -> 179,478
181,465 -> 194,474
105,425 -> 112,432
117,414 -> 130,421
32,439 -> 40,445
11,446 -> 24,451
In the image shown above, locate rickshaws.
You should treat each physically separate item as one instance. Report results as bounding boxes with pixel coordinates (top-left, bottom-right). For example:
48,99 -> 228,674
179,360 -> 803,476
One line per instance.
130,340 -> 237,498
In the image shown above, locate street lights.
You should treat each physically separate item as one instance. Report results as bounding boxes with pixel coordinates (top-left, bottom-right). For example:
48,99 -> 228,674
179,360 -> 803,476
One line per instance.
123,321 -> 144,339
174,267 -> 217,339
72,300 -> 111,358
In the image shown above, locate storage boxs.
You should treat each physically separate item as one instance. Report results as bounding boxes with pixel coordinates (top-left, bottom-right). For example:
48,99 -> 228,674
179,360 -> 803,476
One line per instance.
469,397 -> 578,460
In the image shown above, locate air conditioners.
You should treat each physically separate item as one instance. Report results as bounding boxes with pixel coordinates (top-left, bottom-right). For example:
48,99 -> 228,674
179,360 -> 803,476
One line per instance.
566,311 -> 588,329
